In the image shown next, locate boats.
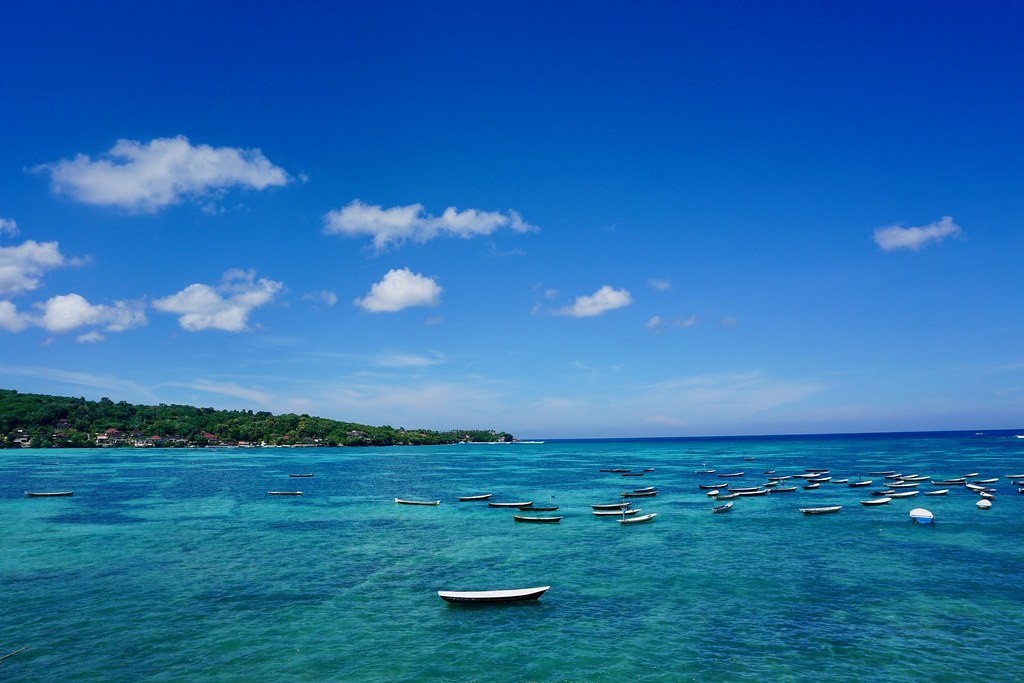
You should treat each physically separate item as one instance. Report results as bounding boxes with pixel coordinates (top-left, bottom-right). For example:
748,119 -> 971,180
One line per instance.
634,487 -> 654,492
976,500 -> 992,509
765,467 -> 1024,499
707,490 -> 719,496
519,507 -> 558,511
769,487 -> 797,492
711,493 -> 739,501
744,457 -> 755,461
438,586 -> 550,603
695,470 -> 716,474
643,468 -> 654,471
289,473 -> 314,477
459,494 -> 492,501
592,509 -> 641,517
859,497 -> 892,506
763,481 -> 778,487
718,473 -> 744,477
514,515 -> 562,524
590,503 -> 630,511
267,491 -> 303,495
620,491 -> 659,498
699,483 -> 728,489
712,502 -> 734,513
616,513 -> 657,525
395,497 -> 440,506
728,487 -> 761,494
737,490 -> 767,497
489,501 -> 533,507
598,469 -> 613,472
613,468 -> 631,473
622,472 -> 644,476
799,506 -> 842,514
25,491 -> 73,497
911,508 -> 935,524
